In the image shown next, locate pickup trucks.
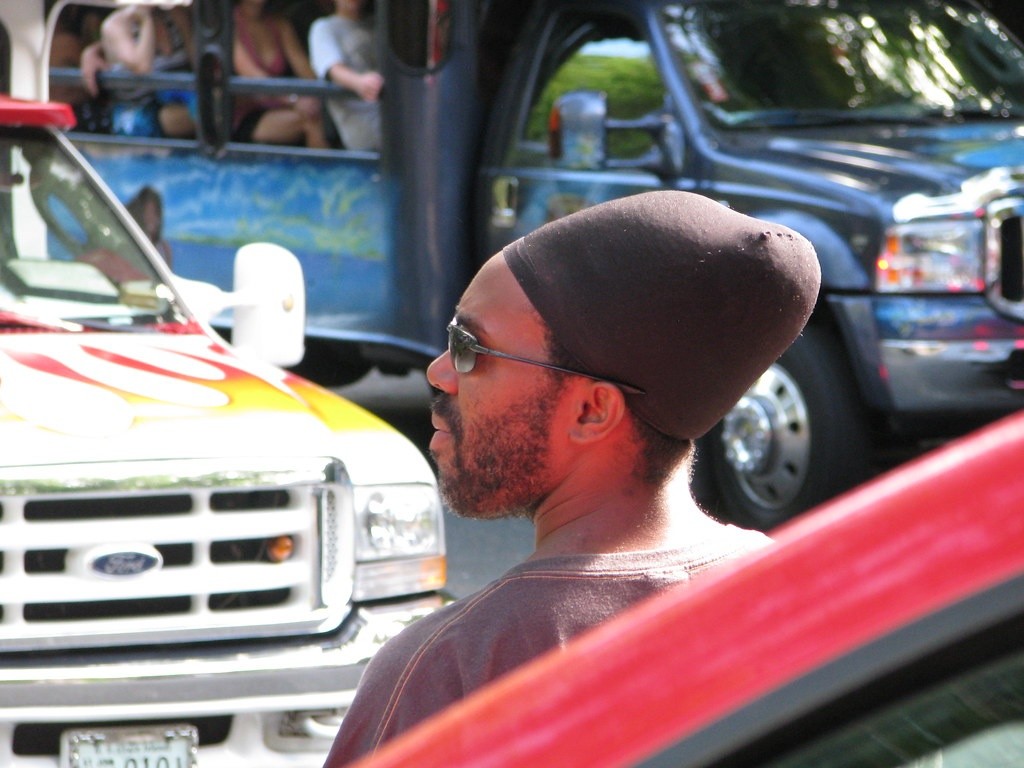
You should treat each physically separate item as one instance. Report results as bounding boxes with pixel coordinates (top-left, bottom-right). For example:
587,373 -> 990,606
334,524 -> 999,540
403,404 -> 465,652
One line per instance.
0,1 -> 1024,541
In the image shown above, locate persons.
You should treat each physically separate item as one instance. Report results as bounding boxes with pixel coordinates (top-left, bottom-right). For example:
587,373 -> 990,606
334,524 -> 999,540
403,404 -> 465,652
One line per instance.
50,0 -> 385,152
322,189 -> 822,768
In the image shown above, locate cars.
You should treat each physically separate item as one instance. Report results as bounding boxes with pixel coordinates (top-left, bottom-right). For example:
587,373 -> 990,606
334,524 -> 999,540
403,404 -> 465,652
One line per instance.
0,101 -> 447,767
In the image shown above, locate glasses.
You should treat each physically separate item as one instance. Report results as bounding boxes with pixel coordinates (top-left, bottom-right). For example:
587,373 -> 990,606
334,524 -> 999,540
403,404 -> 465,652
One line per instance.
446,323 -> 646,396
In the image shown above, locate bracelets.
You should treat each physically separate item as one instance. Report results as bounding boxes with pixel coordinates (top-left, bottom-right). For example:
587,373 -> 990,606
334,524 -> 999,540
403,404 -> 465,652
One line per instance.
288,94 -> 296,109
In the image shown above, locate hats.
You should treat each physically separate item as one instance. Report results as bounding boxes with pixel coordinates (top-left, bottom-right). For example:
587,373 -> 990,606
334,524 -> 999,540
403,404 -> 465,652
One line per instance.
503,188 -> 821,440
